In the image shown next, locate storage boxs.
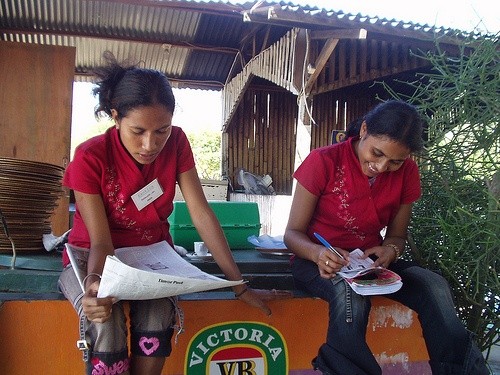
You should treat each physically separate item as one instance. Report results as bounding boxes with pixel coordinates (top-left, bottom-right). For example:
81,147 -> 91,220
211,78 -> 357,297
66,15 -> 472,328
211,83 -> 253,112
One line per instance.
168,199 -> 261,251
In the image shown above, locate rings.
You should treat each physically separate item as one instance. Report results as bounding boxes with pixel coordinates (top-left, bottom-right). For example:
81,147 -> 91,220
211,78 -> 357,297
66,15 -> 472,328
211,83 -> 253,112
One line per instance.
326,260 -> 329,265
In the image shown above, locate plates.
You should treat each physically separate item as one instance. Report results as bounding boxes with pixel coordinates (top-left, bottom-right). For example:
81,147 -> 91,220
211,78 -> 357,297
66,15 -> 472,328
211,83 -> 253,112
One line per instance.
186,253 -> 213,261
255,246 -> 295,258
0,156 -> 66,253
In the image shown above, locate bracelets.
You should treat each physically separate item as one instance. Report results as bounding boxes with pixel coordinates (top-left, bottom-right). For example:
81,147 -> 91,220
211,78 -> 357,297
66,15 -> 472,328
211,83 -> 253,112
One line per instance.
235,288 -> 247,297
82,273 -> 101,290
387,244 -> 400,258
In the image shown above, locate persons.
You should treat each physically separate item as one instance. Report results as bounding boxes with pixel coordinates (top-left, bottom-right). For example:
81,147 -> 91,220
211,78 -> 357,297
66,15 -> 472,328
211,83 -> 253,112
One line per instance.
284,99 -> 490,375
58,52 -> 272,375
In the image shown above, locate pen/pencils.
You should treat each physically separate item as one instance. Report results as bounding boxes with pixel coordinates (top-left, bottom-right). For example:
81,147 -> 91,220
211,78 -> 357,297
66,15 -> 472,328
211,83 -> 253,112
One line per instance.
314,232 -> 352,270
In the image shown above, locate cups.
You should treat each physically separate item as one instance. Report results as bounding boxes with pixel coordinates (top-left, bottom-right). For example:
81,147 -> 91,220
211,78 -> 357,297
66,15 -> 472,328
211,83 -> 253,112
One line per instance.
193,241 -> 209,255
